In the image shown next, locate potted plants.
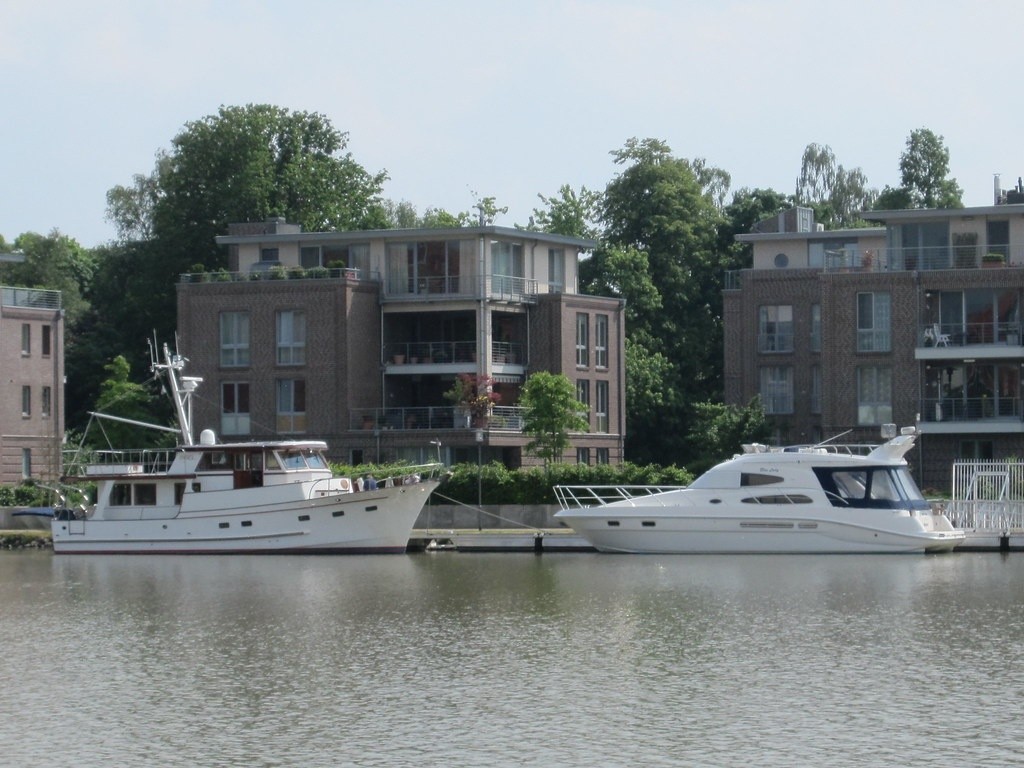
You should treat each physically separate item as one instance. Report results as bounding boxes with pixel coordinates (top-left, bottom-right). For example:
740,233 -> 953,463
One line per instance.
363,413 -> 394,429
838,247 -> 851,272
443,377 -> 510,429
862,250 -> 875,271
981,254 -> 1006,267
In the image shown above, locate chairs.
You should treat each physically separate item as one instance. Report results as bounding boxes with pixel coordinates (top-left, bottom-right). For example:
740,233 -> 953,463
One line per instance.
933,323 -> 952,347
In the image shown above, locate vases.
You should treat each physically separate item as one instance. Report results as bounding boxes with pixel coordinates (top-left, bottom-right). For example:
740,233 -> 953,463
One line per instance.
411,356 -> 418,364
491,354 -> 518,363
393,354 -> 404,364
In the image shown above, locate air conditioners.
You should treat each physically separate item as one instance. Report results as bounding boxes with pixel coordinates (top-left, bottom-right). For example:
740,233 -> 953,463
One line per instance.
812,222 -> 825,232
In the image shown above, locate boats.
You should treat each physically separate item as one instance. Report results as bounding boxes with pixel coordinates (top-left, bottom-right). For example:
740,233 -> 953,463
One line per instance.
49,338 -> 451,557
545,426 -> 965,554
